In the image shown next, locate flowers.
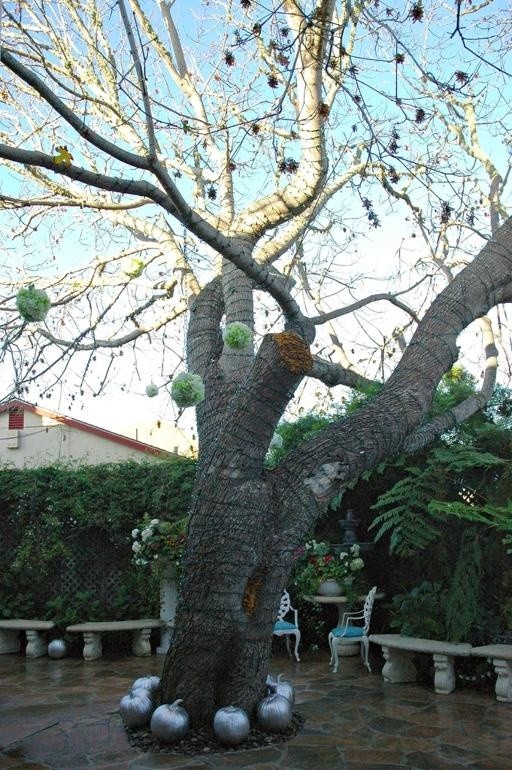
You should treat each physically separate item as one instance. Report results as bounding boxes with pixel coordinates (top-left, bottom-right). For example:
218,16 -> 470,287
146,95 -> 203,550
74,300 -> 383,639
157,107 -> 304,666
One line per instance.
285,538 -> 365,614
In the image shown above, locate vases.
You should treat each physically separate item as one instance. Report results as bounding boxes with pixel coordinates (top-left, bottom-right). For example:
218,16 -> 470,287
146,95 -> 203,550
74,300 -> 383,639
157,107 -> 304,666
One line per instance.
316,578 -> 346,597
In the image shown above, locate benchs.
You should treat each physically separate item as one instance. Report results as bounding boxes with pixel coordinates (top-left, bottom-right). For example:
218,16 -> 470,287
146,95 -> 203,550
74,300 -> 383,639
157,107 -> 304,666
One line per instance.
368,633 -> 512,706
0,619 -> 163,660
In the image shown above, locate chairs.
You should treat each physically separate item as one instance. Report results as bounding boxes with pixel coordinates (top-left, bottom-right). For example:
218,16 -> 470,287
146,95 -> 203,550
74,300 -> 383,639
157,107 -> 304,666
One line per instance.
329,586 -> 377,674
272,589 -> 302,661
